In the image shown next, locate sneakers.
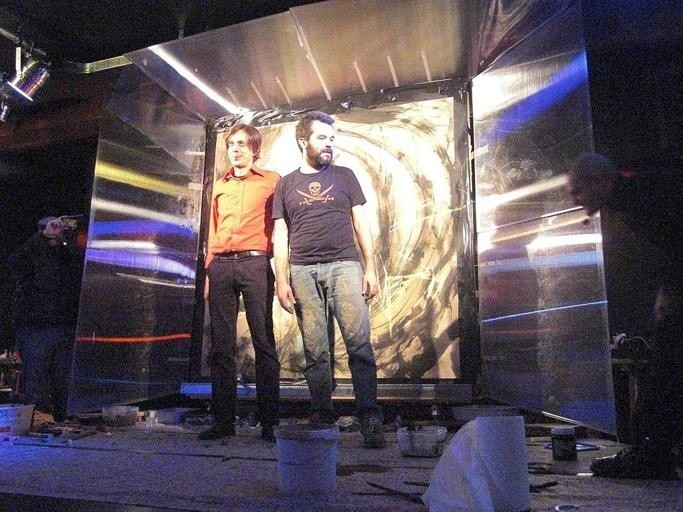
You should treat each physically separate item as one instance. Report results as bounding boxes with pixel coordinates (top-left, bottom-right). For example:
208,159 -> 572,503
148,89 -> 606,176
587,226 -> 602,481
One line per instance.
361,419 -> 384,448
263,425 -> 276,443
589,445 -> 675,479
198,423 -> 235,440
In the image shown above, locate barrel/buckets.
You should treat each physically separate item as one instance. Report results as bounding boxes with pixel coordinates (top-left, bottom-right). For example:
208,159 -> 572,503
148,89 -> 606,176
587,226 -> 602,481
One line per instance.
273,424 -> 340,497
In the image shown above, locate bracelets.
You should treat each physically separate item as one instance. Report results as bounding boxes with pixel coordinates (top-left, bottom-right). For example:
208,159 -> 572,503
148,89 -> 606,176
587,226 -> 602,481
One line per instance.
202,266 -> 208,279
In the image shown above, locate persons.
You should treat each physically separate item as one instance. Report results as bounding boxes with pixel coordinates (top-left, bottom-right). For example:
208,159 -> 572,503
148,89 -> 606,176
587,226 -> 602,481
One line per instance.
2,217 -> 85,423
193,123 -> 283,447
564,149 -> 683,481
269,110 -> 389,450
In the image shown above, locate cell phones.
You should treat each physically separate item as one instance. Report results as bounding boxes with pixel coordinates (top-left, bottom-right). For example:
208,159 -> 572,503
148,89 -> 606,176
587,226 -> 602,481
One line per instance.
61,216 -> 79,229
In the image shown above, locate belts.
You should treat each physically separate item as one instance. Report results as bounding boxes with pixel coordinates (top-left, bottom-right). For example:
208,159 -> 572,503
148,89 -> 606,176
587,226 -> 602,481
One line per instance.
213,250 -> 267,260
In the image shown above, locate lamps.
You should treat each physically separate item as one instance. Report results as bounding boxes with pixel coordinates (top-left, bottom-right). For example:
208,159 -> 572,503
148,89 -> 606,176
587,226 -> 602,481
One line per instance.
6,37 -> 50,102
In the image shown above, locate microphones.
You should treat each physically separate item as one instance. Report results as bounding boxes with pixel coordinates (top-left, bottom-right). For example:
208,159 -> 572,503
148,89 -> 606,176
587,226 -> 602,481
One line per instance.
583,215 -> 590,225
